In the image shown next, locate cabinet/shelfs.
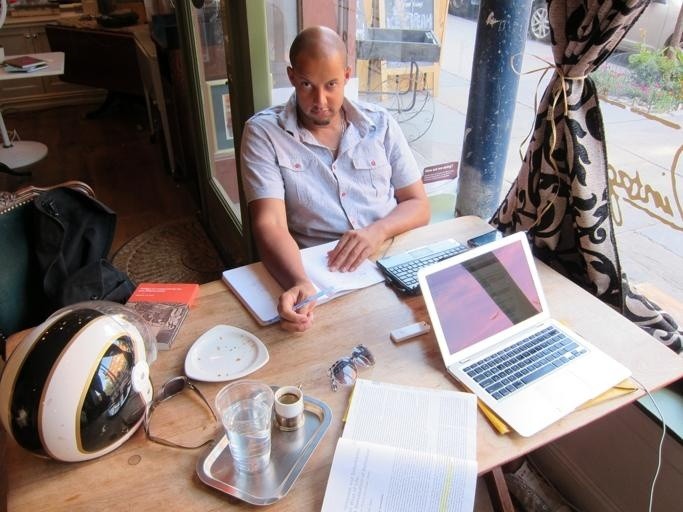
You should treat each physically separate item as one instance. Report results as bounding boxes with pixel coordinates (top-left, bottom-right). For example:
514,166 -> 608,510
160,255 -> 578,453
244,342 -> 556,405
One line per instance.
0,13 -> 177,174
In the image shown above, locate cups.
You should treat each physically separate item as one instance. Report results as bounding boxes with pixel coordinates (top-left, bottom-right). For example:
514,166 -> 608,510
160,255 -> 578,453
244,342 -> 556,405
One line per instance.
273,386 -> 307,434
215,379 -> 274,475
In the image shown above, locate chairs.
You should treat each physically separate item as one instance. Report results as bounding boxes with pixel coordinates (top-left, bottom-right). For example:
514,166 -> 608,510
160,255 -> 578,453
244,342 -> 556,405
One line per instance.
0,180 -> 97,342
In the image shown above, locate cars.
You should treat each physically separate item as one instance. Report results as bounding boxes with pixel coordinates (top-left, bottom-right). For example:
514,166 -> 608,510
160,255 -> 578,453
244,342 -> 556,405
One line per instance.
528,0 -> 682,56
448,0 -> 479,19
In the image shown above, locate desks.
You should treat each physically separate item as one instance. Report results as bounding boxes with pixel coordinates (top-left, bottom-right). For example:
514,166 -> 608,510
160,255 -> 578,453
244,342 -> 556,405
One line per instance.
0,214 -> 683,512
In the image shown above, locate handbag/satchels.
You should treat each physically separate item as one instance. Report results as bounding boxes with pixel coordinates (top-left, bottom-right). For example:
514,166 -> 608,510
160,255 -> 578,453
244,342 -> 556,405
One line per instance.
28,185 -> 133,305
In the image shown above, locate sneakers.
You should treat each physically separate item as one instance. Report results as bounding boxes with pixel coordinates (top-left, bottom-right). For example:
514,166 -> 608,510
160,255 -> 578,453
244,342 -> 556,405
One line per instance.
506,460 -> 571,512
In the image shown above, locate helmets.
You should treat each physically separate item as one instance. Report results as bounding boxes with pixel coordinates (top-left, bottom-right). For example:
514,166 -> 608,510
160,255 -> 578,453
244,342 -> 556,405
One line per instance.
1,300 -> 157,462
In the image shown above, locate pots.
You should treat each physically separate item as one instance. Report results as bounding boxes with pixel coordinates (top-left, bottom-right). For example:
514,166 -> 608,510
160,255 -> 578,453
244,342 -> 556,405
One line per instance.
77,11 -> 138,30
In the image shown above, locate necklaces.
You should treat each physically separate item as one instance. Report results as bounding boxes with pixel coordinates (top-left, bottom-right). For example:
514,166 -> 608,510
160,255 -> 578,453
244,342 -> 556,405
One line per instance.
318,107 -> 347,152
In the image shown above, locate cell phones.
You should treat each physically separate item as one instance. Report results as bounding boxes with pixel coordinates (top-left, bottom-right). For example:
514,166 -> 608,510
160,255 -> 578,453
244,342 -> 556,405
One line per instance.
467,229 -> 506,248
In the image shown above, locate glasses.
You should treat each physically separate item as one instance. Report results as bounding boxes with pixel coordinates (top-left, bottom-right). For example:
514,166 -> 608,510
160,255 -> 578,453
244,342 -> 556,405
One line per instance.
330,344 -> 374,392
143,375 -> 218,449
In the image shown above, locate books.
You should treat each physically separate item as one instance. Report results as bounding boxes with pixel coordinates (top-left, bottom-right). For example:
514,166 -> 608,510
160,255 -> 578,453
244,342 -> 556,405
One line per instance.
320,376 -> 480,510
124,282 -> 199,351
4,52 -> 47,73
221,238 -> 385,326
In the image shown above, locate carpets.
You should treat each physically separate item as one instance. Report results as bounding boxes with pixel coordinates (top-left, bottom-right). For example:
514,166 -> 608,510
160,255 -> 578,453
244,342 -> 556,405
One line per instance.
110,214 -> 227,296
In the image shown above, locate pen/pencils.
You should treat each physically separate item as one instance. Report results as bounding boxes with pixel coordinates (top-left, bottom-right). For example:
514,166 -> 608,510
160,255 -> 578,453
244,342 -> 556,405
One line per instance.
267,283 -> 337,327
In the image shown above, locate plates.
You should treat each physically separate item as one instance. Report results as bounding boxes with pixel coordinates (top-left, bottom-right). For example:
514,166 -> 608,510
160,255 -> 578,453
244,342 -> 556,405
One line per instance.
183,324 -> 270,382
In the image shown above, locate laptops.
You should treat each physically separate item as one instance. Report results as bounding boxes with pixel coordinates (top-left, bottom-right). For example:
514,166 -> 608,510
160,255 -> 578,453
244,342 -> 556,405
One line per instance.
375,231 -> 528,297
417,230 -> 632,437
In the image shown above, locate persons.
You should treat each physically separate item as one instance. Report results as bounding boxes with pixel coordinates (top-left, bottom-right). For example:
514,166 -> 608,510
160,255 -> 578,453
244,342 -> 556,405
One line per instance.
240,25 -> 429,331
